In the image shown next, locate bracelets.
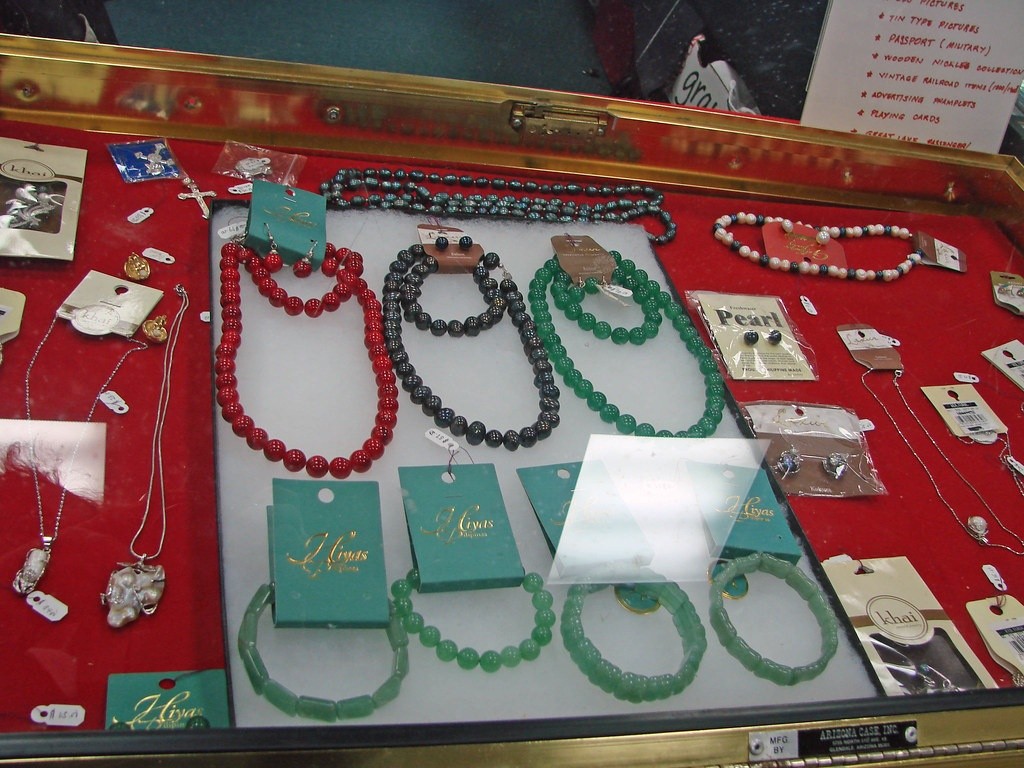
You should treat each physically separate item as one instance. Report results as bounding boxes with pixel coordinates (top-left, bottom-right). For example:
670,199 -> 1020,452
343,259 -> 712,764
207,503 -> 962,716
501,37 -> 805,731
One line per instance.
711,553 -> 837,684
237,581 -> 412,717
391,568 -> 557,673
560,562 -> 708,705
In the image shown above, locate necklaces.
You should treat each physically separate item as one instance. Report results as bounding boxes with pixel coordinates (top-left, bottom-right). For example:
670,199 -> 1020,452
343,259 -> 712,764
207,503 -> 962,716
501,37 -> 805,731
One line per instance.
714,212 -> 927,283
527,251 -> 726,440
319,165 -> 699,245
861,370 -> 1024,554
957,431 -> 1024,497
101,284 -> 190,627
382,245 -> 560,447
11,312 -> 148,595
215,242 -> 398,479
